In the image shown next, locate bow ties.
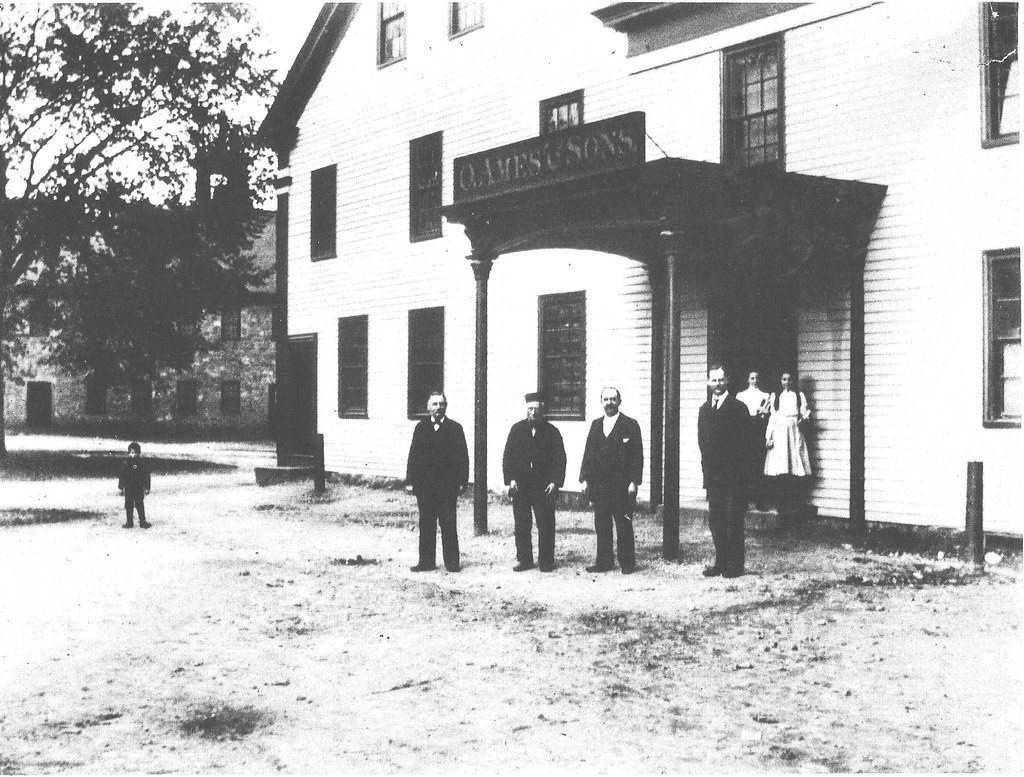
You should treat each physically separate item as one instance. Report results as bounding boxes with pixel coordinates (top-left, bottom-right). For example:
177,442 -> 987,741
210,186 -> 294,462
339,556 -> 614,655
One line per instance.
432,419 -> 442,425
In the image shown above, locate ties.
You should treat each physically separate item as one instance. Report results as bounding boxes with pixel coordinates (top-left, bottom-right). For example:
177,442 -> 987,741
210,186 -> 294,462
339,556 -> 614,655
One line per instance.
712,398 -> 719,410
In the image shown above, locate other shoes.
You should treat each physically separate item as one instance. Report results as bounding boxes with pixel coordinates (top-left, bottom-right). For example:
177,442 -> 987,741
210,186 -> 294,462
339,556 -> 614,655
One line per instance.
540,565 -> 553,571
722,569 -> 744,577
586,565 -> 612,572
410,564 -> 436,572
122,522 -> 133,528
703,566 -> 725,576
622,568 -> 633,574
513,562 -> 534,571
446,565 -> 459,572
140,522 -> 151,528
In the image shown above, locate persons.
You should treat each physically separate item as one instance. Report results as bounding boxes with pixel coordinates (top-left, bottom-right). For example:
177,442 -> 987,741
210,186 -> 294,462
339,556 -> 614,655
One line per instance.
579,386 -> 643,574
402,391 -> 470,573
502,392 -> 567,572
764,368 -> 812,528
696,360 -> 754,579
735,369 -> 772,416
118,442 -> 151,528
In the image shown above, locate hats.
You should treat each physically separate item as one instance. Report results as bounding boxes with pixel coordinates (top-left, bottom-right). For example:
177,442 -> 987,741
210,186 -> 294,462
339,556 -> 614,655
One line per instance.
525,393 -> 545,402
